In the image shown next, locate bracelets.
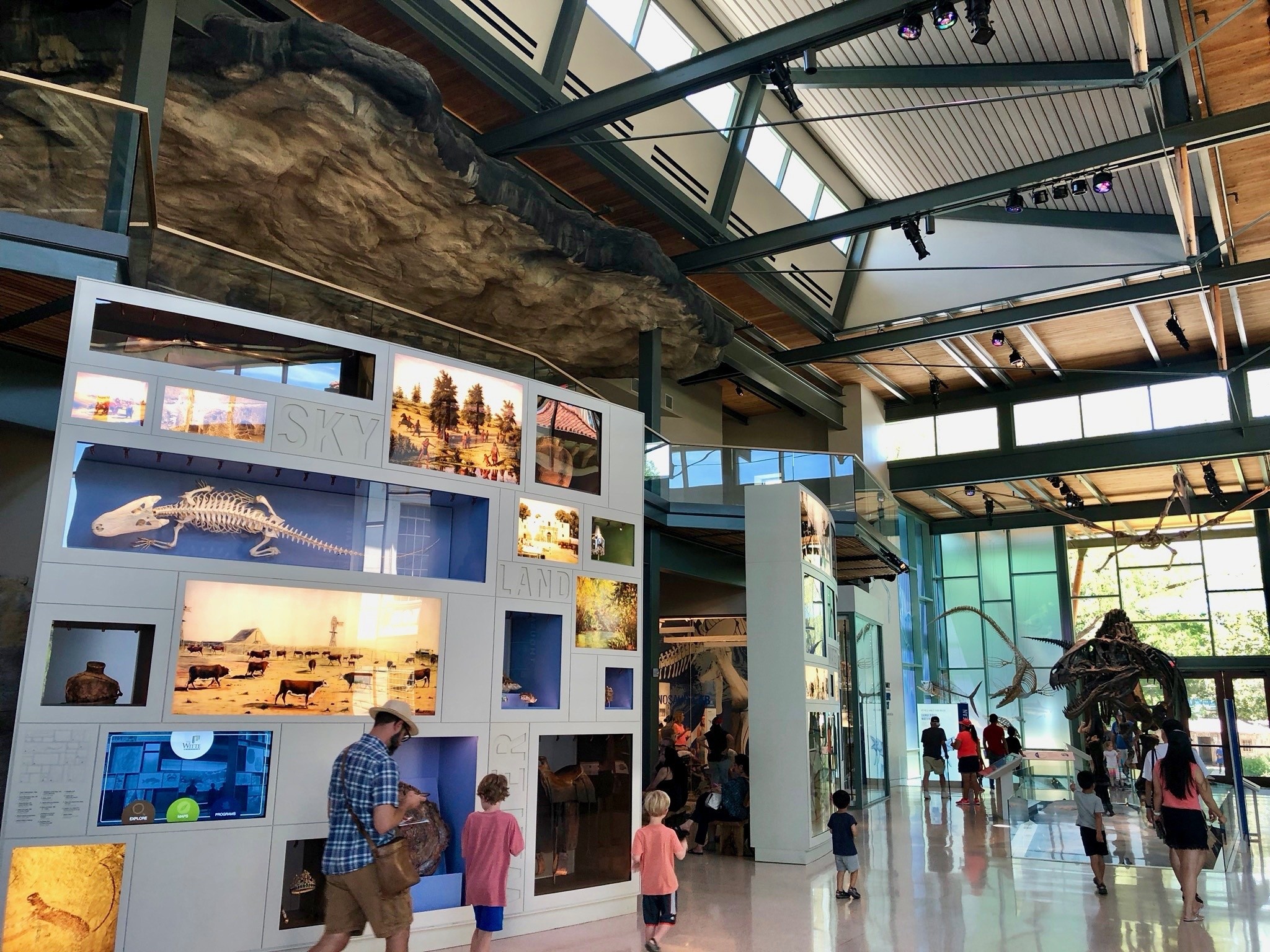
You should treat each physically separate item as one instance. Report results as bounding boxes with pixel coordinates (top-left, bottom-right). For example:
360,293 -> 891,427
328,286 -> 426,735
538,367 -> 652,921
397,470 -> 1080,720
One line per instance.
1154,811 -> 1162,816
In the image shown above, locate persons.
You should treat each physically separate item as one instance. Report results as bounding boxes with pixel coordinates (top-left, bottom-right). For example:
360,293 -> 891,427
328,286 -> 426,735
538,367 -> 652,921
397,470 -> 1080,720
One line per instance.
632,790 -> 689,952
1140,720 -> 1212,901
827,790 -> 861,899
1006,726 -> 1027,770
646,712 -> 749,856
1216,747 -> 1224,773
950,719 -> 980,805
921,716 -> 950,799
983,714 -> 1009,794
308,698 -> 427,952
1070,771 -> 1109,895
1152,725 -> 1227,922
970,738 -> 985,793
1078,709 -> 1142,792
461,774 -> 525,952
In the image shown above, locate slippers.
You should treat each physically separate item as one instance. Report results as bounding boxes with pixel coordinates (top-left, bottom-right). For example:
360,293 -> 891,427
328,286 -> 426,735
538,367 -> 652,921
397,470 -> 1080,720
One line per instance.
669,825 -> 690,835
1194,909 -> 1199,915
687,847 -> 704,855
1184,916 -> 1204,921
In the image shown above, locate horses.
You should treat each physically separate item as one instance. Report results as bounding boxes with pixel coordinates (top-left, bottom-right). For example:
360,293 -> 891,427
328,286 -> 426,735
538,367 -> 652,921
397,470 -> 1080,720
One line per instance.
397,413 -> 414,432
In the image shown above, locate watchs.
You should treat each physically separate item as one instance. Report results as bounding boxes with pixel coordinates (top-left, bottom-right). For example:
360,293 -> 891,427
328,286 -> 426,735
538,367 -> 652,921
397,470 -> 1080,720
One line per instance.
1145,802 -> 1152,808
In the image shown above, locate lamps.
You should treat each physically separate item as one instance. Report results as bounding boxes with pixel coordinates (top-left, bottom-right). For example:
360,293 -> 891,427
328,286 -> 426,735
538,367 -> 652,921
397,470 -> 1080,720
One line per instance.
1009,351 -> 1023,366
898,7 -> 923,41
735,386 -> 743,396
1006,167 -> 1113,214
1050,477 -> 1085,511
758,54 -> 803,115
929,377 -> 942,409
963,0 -> 996,45
891,211 -> 930,261
986,501 -> 993,514
887,551 -> 909,573
1165,300 -> 1191,350
965,486 -> 975,496
1201,460 -> 1229,507
932,0 -> 959,30
991,330 -> 1004,346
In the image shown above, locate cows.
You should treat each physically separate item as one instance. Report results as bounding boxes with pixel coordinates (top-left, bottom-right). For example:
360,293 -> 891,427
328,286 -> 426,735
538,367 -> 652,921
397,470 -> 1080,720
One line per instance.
185,664 -> 230,691
274,679 -> 330,710
185,643 -> 203,655
293,649 -> 431,692
245,661 -> 270,679
247,649 -> 271,662
208,644 -> 225,654
276,651 -> 287,659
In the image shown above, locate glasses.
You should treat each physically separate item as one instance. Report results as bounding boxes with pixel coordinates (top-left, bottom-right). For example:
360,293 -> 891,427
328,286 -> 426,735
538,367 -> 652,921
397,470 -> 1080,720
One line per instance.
394,719 -> 411,743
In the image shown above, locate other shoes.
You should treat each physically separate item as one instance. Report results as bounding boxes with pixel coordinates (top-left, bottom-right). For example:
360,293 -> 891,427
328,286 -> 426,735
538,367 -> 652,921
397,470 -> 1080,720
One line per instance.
990,789 -> 994,793
979,787 -> 984,792
1109,780 -> 1131,790
1182,893 -> 1203,903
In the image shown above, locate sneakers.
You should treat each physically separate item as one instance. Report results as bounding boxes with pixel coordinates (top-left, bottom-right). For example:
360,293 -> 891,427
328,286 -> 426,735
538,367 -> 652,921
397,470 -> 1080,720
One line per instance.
941,791 -> 949,798
955,798 -> 970,805
973,798 -> 980,804
924,790 -> 931,799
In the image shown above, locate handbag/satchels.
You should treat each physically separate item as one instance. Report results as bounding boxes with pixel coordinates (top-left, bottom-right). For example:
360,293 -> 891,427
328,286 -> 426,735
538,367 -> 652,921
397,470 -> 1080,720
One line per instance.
706,792 -> 722,810
372,836 -> 421,898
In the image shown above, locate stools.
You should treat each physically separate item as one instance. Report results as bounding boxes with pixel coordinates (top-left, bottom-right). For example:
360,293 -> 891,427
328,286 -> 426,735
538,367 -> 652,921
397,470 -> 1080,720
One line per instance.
716,820 -> 749,857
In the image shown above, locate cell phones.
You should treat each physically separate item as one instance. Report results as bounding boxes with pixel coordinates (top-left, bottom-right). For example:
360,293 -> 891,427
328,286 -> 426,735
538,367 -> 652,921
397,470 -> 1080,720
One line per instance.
418,792 -> 430,797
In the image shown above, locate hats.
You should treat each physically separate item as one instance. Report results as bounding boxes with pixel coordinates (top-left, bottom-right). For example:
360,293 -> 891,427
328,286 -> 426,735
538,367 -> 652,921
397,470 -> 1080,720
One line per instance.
712,717 -> 724,725
931,716 -> 939,723
958,719 -> 971,727
369,699 -> 419,736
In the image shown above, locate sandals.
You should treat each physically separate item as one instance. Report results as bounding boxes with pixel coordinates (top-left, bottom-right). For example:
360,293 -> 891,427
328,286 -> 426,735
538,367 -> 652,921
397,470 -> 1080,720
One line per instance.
1093,877 -> 1098,885
1097,883 -> 1107,895
835,890 -> 850,898
847,887 -> 861,899
644,938 -> 661,952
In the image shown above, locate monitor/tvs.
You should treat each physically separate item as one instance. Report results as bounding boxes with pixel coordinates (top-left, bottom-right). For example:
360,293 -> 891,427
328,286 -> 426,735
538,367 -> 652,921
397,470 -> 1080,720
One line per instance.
982,755 -> 1018,774
